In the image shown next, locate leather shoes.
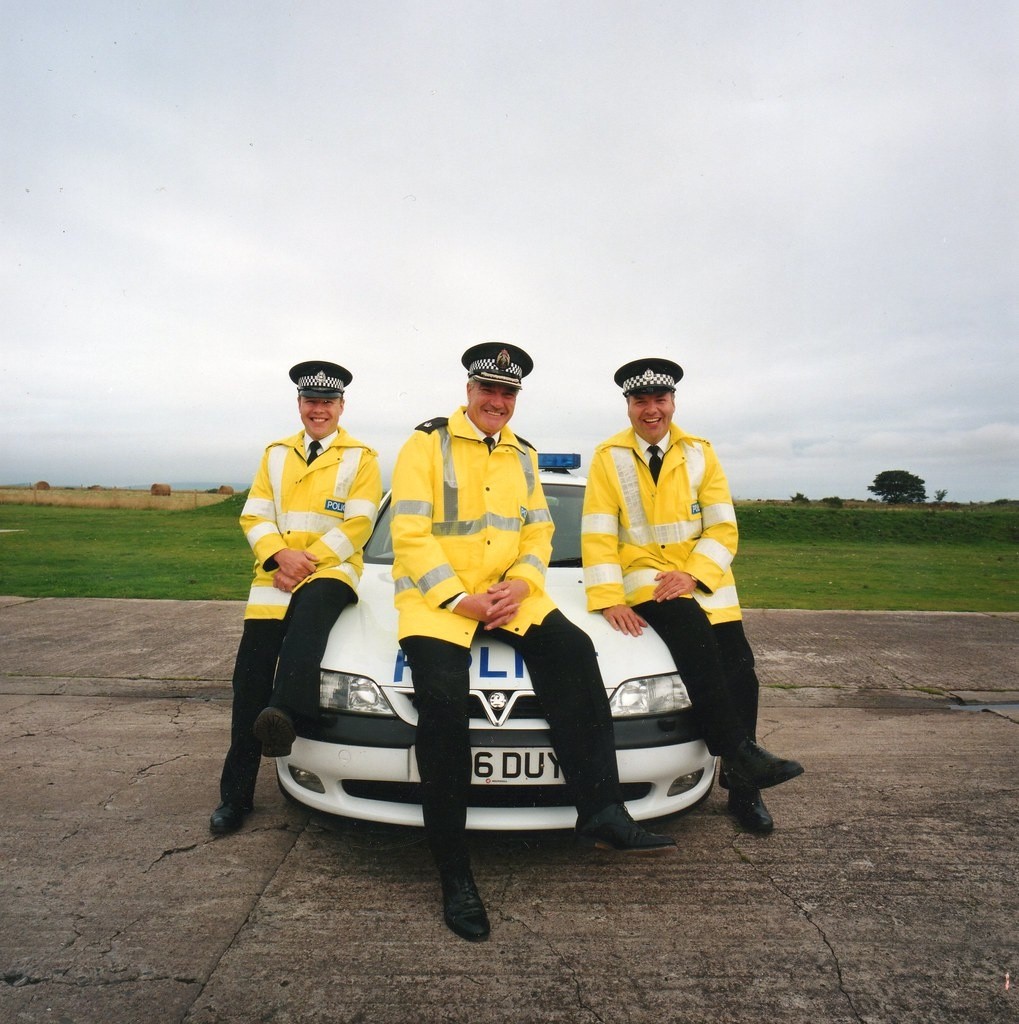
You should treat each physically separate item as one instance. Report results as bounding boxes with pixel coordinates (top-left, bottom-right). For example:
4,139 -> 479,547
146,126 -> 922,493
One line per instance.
728,787 -> 772,833
571,806 -> 677,856
210,800 -> 252,832
441,868 -> 490,941
253,707 -> 296,757
733,738 -> 805,788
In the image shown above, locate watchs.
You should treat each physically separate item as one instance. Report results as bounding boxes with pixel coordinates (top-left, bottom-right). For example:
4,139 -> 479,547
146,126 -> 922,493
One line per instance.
691,576 -> 698,583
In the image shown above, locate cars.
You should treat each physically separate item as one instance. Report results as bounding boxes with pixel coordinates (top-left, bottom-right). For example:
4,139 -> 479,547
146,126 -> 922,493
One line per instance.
271,452 -> 721,834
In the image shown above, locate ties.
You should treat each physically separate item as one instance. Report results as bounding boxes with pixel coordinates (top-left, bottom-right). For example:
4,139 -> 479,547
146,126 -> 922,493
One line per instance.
483,437 -> 494,454
646,446 -> 663,486
306,440 -> 320,466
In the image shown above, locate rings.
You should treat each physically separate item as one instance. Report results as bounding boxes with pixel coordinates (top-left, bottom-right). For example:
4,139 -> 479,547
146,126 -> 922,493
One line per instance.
280,588 -> 285,591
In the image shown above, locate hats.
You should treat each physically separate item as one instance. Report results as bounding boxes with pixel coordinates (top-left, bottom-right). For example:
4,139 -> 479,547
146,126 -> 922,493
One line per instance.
614,358 -> 684,396
289,361 -> 352,399
461,342 -> 533,390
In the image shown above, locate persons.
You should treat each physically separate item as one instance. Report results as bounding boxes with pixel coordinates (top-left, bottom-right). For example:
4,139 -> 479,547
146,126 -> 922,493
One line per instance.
207,360 -> 383,836
578,357 -> 805,837
390,341 -> 679,944
